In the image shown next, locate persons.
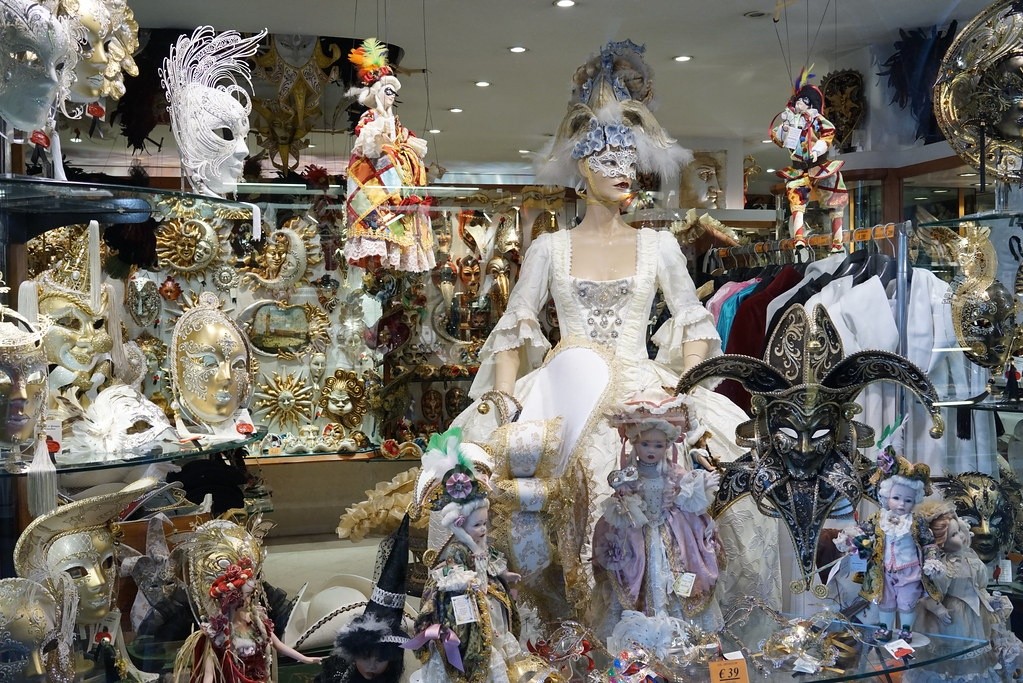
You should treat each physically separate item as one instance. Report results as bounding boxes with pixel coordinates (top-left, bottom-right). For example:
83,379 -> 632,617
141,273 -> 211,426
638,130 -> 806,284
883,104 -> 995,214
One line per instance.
826,444 -> 1023,683
256,350 -> 367,430
343,38 -> 437,274
411,427 -> 524,682
314,511 -> 411,682
175,510 -> 322,683
770,59 -> 848,263
422,39 -> 804,612
214,266 -> 237,290
238,217 -> 324,293
156,219 -> 220,273
592,390 -> 724,648
161,275 -> 184,302
341,318 -> 364,355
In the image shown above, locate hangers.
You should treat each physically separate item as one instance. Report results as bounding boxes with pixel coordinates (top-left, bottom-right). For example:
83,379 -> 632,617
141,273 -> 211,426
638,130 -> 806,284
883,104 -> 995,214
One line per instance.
711,222 -> 913,289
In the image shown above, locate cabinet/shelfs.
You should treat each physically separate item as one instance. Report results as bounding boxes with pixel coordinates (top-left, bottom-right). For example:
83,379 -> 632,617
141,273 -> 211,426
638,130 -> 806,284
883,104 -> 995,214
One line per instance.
919,212 -> 1022,641
0,177 -> 254,476
